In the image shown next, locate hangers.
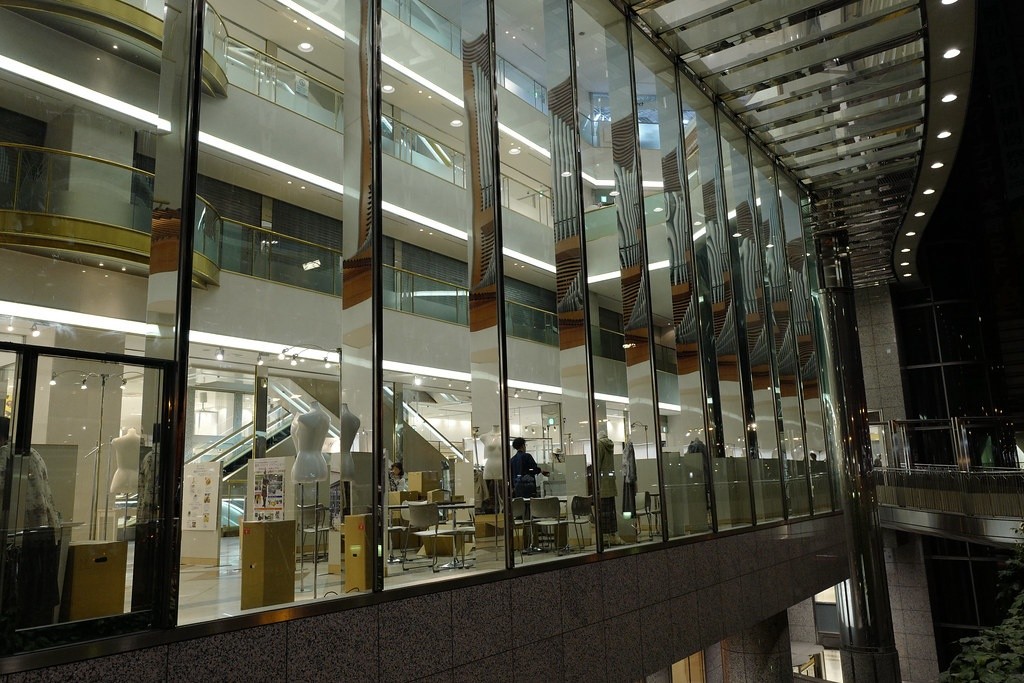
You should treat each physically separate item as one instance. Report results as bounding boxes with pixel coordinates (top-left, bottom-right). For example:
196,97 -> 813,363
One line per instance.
473,464 -> 484,473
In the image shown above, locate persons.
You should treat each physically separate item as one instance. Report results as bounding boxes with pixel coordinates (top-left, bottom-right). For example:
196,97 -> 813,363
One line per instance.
510,437 -> 551,552
874,454 -> 883,467
289,400 -> 331,483
810,452 -> 817,461
341,403 -> 361,481
478,424 -> 506,480
596,430 -> 625,548
109,428 -> 142,493
685,437 -> 713,530
0,416 -> 63,629
387,462 -> 410,493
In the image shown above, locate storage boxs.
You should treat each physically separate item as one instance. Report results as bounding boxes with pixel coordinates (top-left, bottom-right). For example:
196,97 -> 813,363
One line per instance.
389,491 -> 418,525
408,471 -> 440,501
451,495 -> 464,502
476,513 -> 504,538
426,489 -> 451,504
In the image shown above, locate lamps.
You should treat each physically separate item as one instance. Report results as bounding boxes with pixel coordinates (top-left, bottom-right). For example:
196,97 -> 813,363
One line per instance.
323,358 -> 331,368
537,392 -> 542,400
525,426 -> 528,432
553,427 -> 556,431
278,347 -> 288,360
256,352 -> 264,365
291,353 -> 298,365
514,389 -> 521,399
214,347 -> 224,360
532,429 -> 535,434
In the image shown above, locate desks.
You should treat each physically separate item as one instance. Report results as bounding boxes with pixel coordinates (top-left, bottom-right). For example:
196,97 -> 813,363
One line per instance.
516,497 -> 581,552
366,504 -> 475,572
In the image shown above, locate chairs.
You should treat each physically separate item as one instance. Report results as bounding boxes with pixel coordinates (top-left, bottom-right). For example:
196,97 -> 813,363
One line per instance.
529,497 -> 568,556
635,493 -> 650,537
296,503 -> 329,563
402,503 -> 466,573
450,499 -> 475,549
560,495 -> 597,554
635,492 -> 654,543
511,497 -> 540,564
